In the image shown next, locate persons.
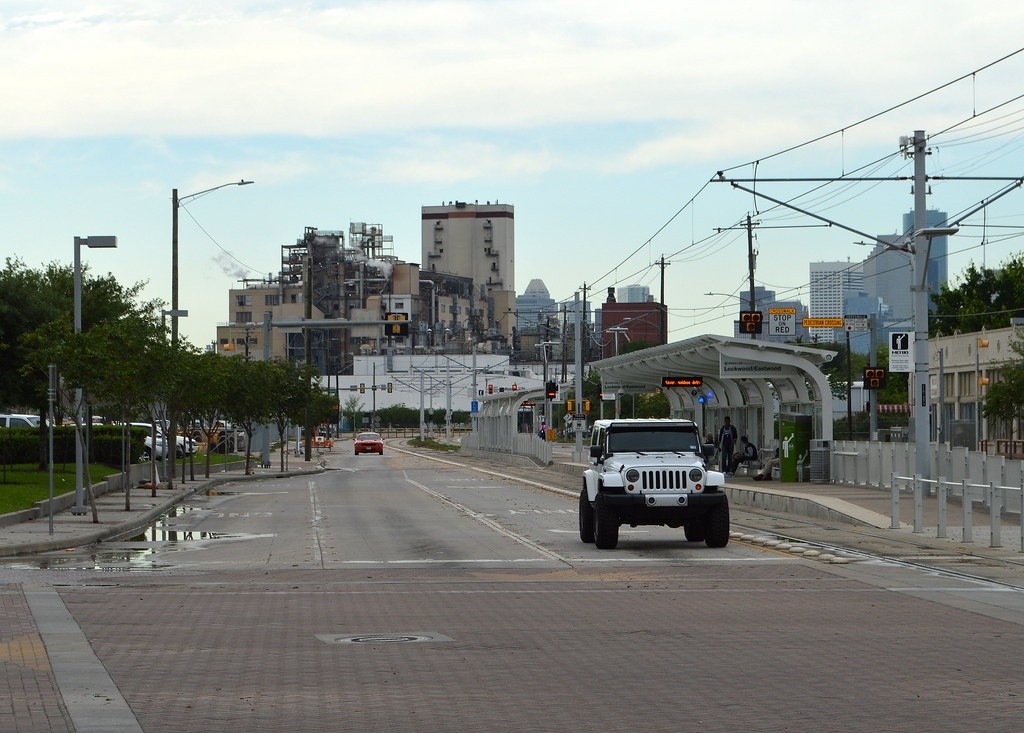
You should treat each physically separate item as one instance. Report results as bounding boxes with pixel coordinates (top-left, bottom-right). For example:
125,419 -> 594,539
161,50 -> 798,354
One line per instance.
718,416 -> 738,474
705,432 -> 721,453
724,436 -> 757,477
540,422 -> 548,440
752,447 -> 779,481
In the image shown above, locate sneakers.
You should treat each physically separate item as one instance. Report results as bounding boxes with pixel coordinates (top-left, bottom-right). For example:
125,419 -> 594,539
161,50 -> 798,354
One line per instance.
726,472 -> 736,478
725,472 -> 730,477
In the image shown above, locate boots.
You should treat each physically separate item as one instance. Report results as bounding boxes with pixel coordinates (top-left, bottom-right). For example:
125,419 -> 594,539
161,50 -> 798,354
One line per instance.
763,473 -> 772,480
753,474 -> 764,481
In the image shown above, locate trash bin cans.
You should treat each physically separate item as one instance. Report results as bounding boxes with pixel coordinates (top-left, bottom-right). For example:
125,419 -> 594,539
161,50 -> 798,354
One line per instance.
809,439 -> 830,483
777,412 -> 813,483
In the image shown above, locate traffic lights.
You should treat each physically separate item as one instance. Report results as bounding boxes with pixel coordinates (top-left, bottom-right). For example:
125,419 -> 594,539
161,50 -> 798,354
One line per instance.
979,377 -> 990,386
740,311 -> 763,334
863,367 -> 886,390
978,335 -> 989,348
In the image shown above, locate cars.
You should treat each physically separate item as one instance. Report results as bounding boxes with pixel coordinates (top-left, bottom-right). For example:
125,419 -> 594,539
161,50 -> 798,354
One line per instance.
0,413 -> 231,464
352,431 -> 385,456
318,427 -> 332,436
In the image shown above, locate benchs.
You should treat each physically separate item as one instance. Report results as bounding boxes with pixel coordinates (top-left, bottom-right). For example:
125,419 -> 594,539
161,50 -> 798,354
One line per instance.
708,448 -> 718,465
742,448 -> 761,475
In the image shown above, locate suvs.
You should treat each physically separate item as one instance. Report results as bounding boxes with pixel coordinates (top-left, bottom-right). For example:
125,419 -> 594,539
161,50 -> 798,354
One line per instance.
578,418 -> 730,550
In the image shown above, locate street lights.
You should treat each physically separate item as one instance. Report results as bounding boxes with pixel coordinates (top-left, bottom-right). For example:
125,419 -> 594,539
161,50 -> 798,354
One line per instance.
534,341 -> 561,463
704,293 -> 757,340
165,180 -> 256,479
160,309 -> 189,482
66,234 -> 118,515
882,225 -> 961,497
